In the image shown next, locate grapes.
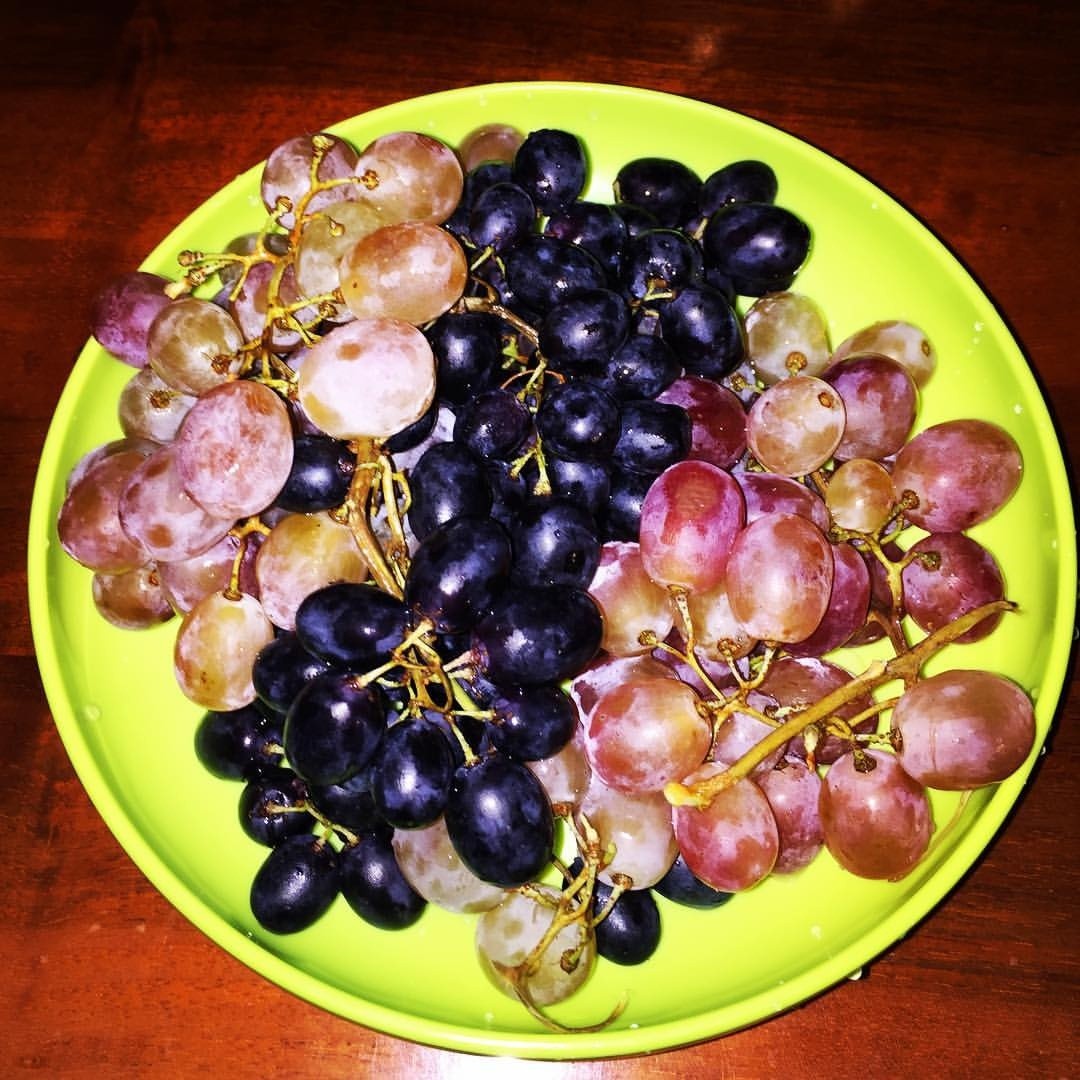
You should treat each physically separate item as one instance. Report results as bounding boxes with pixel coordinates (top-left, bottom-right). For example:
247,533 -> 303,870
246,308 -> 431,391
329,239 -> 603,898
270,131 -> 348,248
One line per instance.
50,123 -> 524,718
386,288 -> 1038,1011
191,126 -> 814,967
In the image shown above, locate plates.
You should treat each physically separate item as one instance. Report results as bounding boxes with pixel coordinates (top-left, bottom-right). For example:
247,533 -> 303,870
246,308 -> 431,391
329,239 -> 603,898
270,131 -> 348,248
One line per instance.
28,78 -> 1076,1062
